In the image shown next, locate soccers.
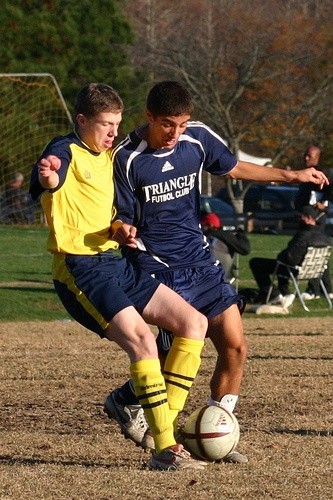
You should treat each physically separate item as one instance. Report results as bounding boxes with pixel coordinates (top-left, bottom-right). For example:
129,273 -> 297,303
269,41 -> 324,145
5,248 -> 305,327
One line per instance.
182,403 -> 241,463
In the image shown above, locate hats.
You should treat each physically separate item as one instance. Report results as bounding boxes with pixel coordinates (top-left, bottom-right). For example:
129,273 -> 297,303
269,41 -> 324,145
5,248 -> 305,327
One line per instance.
201,213 -> 220,229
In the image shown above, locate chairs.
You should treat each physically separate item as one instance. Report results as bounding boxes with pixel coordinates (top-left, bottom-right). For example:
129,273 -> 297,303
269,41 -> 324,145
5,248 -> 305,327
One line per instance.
265,245 -> 333,312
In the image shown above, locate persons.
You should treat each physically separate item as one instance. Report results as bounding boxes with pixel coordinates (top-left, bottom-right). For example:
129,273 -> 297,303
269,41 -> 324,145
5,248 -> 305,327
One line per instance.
247,204 -> 328,314
2,171 -> 36,224
35,81 -> 213,473
297,145 -> 333,301
105,80 -> 329,465
199,212 -> 250,280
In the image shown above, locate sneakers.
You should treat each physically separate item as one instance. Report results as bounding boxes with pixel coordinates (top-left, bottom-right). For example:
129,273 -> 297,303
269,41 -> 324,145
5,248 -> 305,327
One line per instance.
269,291 -> 332,308
225,450 -> 248,463
103,389 -> 149,449
140,429 -> 155,451
149,445 -> 205,470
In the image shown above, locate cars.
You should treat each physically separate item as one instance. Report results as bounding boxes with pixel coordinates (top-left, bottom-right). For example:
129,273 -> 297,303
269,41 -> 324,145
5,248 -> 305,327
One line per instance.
217,185 -> 333,234
200,195 -> 239,236
